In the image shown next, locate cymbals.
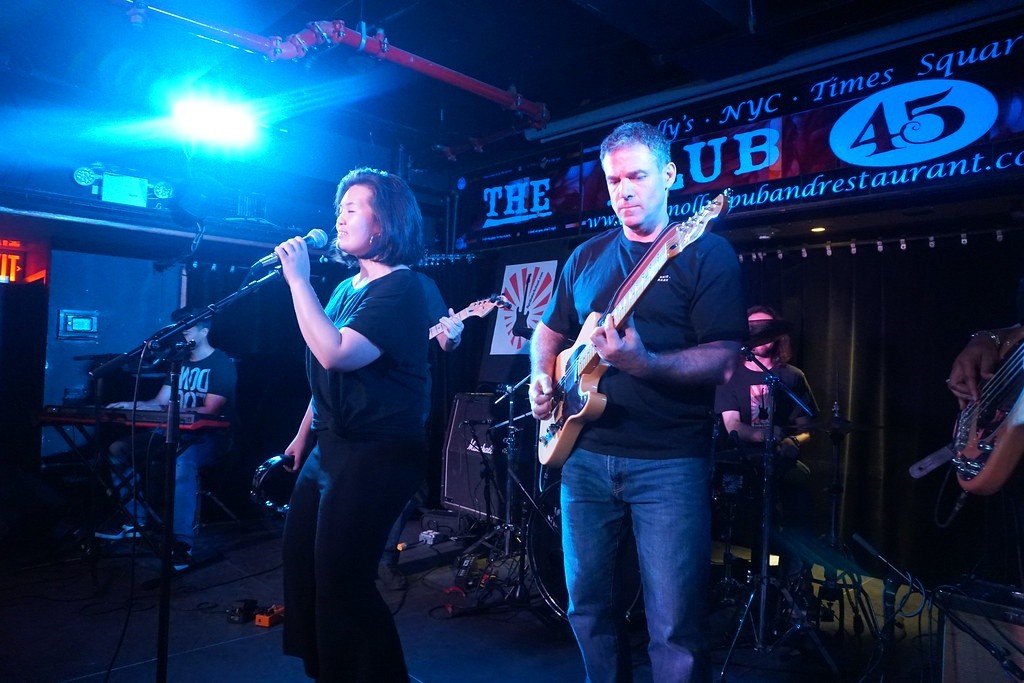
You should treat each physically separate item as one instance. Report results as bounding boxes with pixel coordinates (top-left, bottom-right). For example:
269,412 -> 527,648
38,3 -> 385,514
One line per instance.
250,453 -> 302,515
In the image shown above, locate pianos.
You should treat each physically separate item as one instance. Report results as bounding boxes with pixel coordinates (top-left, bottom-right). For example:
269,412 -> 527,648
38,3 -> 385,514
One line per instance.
37,404 -> 230,568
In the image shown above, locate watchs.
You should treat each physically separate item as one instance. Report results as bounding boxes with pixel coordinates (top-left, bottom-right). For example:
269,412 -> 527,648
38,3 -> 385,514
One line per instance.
790,436 -> 800,448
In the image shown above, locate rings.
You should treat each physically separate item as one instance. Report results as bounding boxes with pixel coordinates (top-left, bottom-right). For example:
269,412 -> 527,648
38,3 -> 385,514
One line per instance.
945,379 -> 950,383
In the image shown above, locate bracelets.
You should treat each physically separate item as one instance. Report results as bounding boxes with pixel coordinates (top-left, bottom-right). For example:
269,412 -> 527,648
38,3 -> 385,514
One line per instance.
971,331 -> 1000,345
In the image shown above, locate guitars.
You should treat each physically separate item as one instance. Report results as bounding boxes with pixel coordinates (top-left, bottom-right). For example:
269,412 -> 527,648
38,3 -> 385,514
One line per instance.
951,322 -> 1023,496
538,186 -> 732,470
429,293 -> 513,340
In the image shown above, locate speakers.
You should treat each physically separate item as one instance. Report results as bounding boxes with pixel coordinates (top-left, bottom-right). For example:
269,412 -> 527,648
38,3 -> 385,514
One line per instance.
934,576 -> 1024,683
441,393 -> 550,529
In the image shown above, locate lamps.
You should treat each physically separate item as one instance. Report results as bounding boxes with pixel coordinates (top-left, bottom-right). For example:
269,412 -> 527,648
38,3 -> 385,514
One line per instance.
74,163 -> 175,207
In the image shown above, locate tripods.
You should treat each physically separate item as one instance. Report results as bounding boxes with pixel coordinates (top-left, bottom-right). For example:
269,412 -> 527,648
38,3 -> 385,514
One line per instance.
715,347 -> 888,683
459,376 -> 568,640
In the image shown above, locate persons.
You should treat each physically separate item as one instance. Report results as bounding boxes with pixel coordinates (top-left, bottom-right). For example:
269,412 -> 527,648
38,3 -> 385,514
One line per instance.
527,122 -> 750,683
376,271 -> 465,589
274,168 -> 430,683
945,278 -> 1024,590
714,304 -> 834,621
92,304 -> 236,571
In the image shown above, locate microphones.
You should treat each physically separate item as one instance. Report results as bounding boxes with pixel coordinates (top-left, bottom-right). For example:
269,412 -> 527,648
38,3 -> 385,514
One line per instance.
250,229 -> 328,271
464,418 -> 492,425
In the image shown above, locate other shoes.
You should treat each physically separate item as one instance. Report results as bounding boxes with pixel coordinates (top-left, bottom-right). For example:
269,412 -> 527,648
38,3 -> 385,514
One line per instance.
94,521 -> 145,540
806,594 -> 834,623
170,540 -> 193,570
